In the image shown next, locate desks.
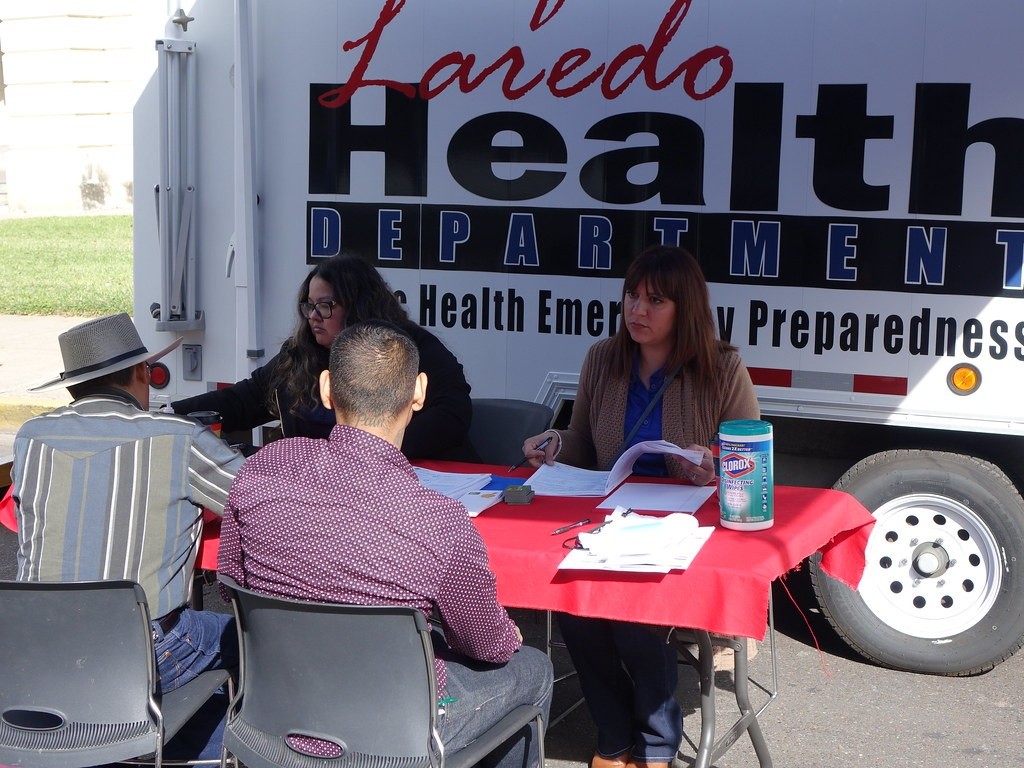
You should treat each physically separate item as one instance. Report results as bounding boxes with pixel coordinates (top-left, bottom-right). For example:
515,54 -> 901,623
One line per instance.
11,461 -> 852,768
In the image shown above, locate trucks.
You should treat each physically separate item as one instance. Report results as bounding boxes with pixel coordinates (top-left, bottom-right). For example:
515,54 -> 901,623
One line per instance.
134,0 -> 1023,675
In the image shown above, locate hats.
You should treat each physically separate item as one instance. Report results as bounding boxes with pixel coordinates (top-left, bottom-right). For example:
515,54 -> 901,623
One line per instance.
31,312 -> 183,391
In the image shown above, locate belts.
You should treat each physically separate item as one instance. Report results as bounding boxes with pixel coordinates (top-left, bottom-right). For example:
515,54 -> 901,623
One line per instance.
152,602 -> 190,643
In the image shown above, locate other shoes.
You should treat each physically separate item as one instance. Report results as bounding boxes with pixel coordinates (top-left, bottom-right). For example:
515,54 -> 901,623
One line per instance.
626,758 -> 672,768
588,750 -> 632,768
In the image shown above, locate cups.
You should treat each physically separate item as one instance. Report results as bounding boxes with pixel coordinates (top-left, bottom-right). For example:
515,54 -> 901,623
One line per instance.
188,410 -> 223,436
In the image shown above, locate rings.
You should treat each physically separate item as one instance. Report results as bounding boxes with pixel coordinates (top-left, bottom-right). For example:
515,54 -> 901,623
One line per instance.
690,474 -> 697,480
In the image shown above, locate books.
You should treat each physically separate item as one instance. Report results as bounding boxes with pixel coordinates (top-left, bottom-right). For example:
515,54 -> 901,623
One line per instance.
412,465 -> 504,518
522,439 -> 705,498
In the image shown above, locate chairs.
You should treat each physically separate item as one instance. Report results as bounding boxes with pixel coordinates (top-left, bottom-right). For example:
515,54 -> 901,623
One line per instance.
216,571 -> 545,768
449,398 -> 554,467
0,580 -> 234,768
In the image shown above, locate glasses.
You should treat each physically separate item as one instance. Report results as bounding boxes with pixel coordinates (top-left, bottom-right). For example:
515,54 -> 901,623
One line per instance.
299,298 -> 348,318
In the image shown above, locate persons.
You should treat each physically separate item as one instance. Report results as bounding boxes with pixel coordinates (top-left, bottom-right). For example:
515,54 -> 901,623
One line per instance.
214,318 -> 553,768
7,313 -> 247,768
157,252 -> 472,463
520,246 -> 763,768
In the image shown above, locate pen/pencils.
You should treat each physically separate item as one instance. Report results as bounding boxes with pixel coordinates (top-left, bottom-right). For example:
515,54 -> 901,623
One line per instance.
551,519 -> 590,535
508,437 -> 553,473
